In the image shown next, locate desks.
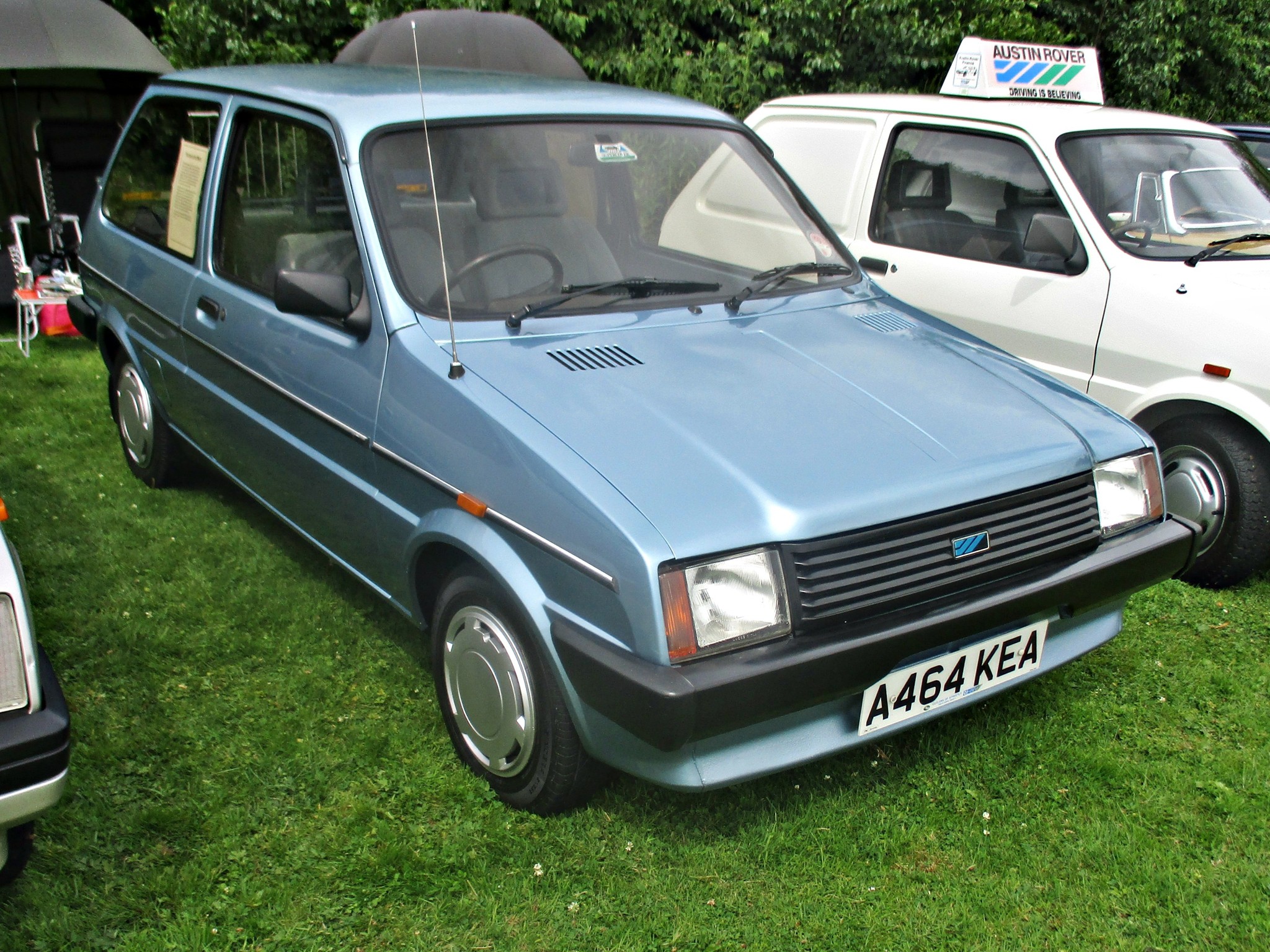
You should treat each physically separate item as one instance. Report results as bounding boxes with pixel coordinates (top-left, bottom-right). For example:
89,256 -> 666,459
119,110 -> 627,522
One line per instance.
14,286 -> 82,360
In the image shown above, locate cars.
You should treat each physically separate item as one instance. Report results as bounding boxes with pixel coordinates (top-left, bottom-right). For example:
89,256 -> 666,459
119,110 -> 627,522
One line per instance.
655,38 -> 1270,591
0,514 -> 75,889
70,61 -> 1203,823
1213,124 -> 1270,184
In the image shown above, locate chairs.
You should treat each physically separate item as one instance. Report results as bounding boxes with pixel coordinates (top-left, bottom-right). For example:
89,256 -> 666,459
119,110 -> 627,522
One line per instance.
991,152 -> 1118,273
276,163 -> 466,316
880,156 -> 993,263
468,150 -> 631,306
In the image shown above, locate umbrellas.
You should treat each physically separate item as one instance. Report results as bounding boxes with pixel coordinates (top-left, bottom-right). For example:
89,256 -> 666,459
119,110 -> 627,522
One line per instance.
333,7 -> 590,80
1,0 -> 176,273
